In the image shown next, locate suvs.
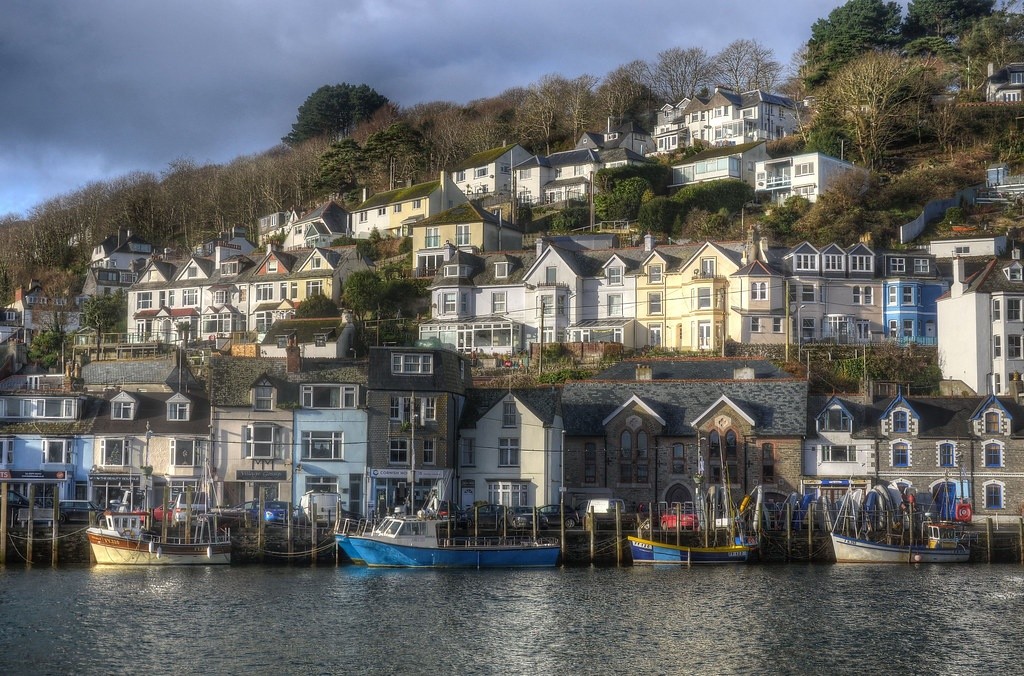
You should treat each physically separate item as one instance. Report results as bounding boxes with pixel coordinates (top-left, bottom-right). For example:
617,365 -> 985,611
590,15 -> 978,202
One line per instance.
535,503 -> 580,529
57,500 -> 110,524
410,499 -> 462,522
460,504 -> 508,529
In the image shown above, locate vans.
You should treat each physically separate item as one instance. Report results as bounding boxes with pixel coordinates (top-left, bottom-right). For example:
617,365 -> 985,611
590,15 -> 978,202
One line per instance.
172,490 -> 210,526
296,490 -> 342,526
223,499 -> 293,526
576,498 -> 628,527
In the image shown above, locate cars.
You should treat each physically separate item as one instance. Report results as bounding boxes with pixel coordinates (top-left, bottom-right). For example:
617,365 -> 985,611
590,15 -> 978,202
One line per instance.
710,504 -> 741,527
6,489 -> 48,522
505,505 -> 549,529
341,508 -> 365,526
742,502 -> 783,528
662,507 -> 700,529
136,501 -> 177,523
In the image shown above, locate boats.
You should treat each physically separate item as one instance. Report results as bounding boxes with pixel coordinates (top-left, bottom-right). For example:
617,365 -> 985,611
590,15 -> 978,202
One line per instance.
252,535 -> 339,562
85,514 -> 233,566
330,510 -> 562,569
625,502 -> 762,565
829,475 -> 972,563
